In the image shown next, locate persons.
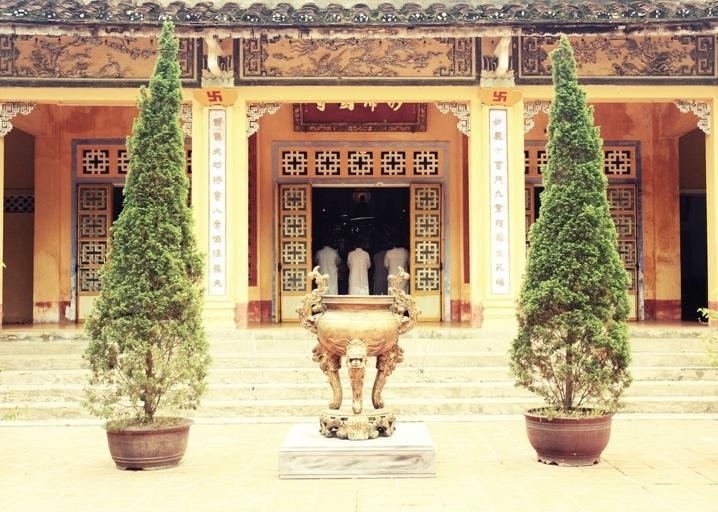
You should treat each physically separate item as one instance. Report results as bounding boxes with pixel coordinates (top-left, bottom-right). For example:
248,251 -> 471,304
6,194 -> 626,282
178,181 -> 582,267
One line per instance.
315,237 -> 410,295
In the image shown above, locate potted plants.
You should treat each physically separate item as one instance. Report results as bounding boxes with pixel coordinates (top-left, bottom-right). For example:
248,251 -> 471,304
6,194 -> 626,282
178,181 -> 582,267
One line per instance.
82,18 -> 213,471
508,32 -> 635,469
